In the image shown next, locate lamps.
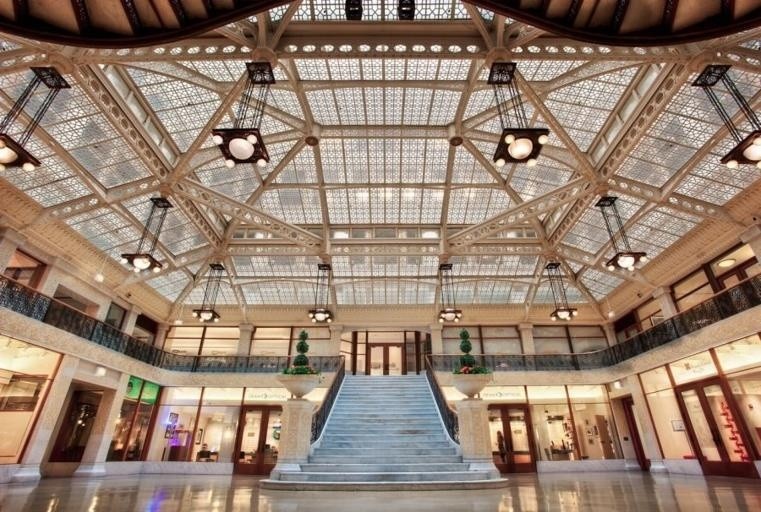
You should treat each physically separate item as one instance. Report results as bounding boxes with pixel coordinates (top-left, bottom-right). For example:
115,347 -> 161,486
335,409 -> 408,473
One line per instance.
193,262 -> 225,324
487,62 -> 550,168
119,197 -> 173,275
0,66 -> 71,173
437,263 -> 462,324
544,262 -> 578,322
692,64 -> 761,171
210,61 -> 277,169
595,196 -> 648,272
308,264 -> 333,325
716,258 -> 736,268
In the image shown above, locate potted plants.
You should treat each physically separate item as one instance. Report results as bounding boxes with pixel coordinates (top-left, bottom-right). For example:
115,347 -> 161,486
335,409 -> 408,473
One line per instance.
275,329 -> 319,401
449,327 -> 494,401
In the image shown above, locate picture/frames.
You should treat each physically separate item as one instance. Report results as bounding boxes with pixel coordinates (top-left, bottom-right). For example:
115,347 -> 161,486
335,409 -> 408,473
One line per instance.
649,314 -> 664,327
